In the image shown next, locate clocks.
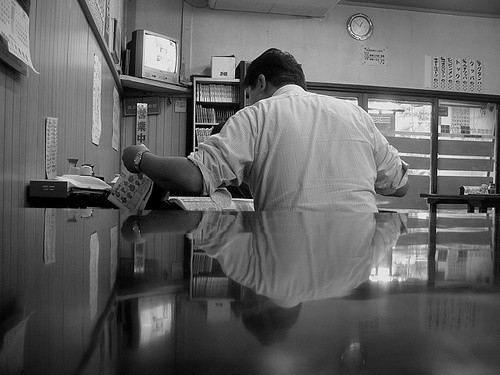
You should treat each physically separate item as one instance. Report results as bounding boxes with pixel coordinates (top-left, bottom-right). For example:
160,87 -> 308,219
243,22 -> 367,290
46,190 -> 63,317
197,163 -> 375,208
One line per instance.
347,13 -> 373,41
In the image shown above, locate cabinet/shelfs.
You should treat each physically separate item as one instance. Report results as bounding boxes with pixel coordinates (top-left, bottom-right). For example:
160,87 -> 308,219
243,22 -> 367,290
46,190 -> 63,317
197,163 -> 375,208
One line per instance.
185,76 -> 247,156
184,234 -> 242,301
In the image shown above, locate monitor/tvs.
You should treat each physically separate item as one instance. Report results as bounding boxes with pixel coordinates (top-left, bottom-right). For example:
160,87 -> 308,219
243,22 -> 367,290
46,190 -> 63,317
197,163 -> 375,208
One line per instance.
125,29 -> 180,84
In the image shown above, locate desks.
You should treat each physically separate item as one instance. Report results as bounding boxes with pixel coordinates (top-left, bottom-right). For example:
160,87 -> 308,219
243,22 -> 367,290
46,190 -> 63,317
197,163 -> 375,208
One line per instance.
420,193 -> 500,213
0,209 -> 500,374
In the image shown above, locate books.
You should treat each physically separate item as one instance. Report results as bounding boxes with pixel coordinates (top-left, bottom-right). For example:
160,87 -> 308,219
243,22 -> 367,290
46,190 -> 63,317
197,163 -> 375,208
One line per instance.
193,239 -> 229,298
195,83 -> 240,147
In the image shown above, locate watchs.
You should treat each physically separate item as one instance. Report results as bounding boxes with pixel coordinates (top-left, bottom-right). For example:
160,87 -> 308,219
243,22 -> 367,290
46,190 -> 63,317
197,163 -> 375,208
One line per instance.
132,218 -> 142,239
133,149 -> 149,175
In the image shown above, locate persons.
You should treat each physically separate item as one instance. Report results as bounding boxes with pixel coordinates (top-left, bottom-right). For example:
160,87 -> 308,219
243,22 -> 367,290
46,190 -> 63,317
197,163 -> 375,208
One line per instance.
121,48 -> 409,211
122,212 -> 409,344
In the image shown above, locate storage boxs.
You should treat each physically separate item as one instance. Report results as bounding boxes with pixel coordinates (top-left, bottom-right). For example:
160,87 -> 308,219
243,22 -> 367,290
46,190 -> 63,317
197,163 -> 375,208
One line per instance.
211,56 -> 235,79
206,301 -> 230,322
